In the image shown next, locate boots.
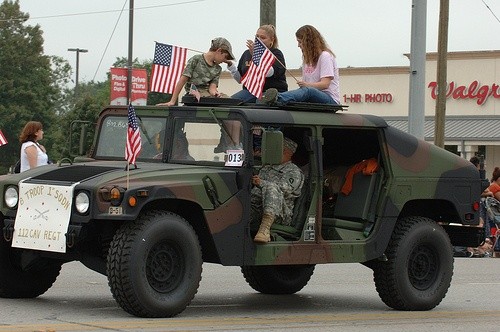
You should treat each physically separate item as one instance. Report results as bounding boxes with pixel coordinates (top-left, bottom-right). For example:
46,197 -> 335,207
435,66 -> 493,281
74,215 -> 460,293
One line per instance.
254,211 -> 276,242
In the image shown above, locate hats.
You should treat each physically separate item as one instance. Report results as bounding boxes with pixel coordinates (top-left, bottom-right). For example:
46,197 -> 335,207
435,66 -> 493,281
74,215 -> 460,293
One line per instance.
211,37 -> 236,60
283,137 -> 298,153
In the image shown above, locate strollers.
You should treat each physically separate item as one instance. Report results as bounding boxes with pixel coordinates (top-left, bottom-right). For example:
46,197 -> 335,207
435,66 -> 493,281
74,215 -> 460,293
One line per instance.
480,196 -> 500,257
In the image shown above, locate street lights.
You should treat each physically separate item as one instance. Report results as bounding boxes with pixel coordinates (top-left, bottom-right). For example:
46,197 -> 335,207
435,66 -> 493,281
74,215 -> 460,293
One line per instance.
66,48 -> 88,88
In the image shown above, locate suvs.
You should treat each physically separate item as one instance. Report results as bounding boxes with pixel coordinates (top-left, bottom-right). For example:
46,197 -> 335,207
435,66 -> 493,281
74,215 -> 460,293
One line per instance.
0,87 -> 491,318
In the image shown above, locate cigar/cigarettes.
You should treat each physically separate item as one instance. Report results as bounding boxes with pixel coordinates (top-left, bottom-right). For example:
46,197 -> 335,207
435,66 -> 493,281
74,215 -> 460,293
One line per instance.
154,153 -> 162,159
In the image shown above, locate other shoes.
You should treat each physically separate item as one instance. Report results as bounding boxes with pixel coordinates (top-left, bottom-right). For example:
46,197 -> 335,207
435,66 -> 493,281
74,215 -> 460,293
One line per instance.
256,88 -> 278,106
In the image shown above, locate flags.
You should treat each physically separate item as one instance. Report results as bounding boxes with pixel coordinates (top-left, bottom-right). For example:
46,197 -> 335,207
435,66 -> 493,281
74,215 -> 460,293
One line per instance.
125,103 -> 142,164
150,43 -> 187,94
0,130 -> 8,146
240,37 -> 275,100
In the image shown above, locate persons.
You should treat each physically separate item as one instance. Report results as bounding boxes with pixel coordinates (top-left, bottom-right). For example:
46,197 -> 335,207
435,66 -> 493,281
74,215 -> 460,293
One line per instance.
20,121 -> 48,173
448,156 -> 500,258
250,136 -> 305,242
260,25 -> 342,105
214,24 -> 289,152
158,129 -> 195,161
156,24 -> 236,106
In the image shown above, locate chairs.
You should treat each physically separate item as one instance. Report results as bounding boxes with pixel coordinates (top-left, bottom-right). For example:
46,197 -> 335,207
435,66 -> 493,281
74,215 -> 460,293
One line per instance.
486,197 -> 500,249
250,180 -> 307,239
323,167 -> 383,230
